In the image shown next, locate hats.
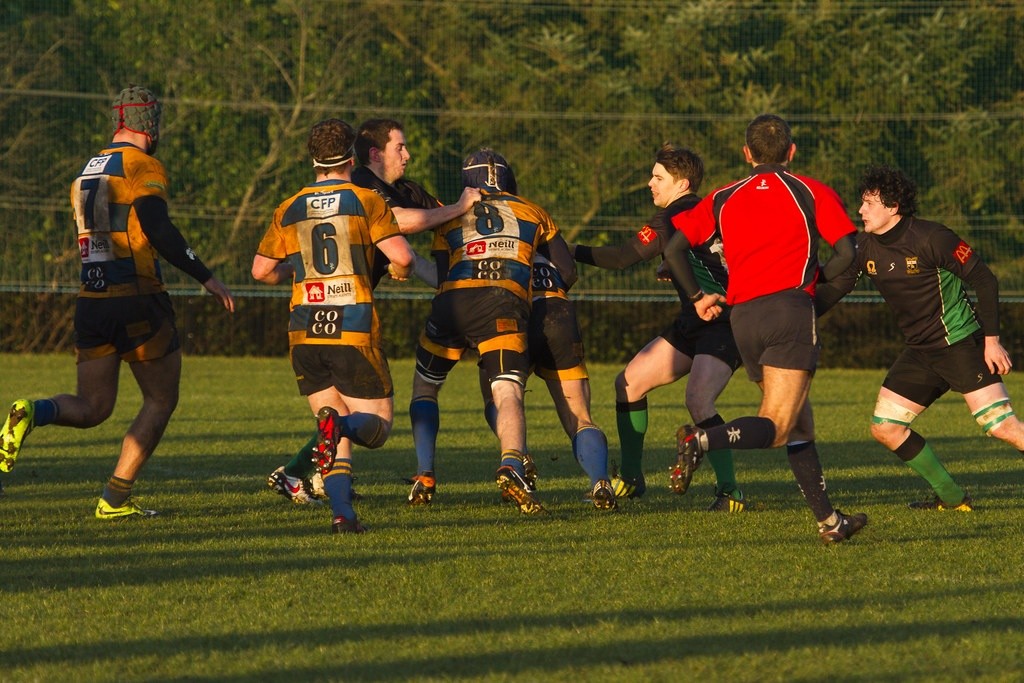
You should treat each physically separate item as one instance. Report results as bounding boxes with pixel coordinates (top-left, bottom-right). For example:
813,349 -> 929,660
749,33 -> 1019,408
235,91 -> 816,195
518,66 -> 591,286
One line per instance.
112,83 -> 161,156
462,150 -> 517,197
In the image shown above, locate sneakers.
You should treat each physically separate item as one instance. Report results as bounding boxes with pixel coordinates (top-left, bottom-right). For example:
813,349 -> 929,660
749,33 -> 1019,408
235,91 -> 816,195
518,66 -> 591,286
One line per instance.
266,466 -> 322,506
581,459 -> 646,499
332,516 -> 367,534
591,479 -> 619,511
501,454 -> 538,505
96,494 -> 157,521
311,407 -> 343,476
817,509 -> 868,547
0,398 -> 36,472
707,486 -> 747,513
323,475 -> 366,502
906,489 -> 975,513
669,425 -> 705,496
495,465 -> 550,517
402,471 -> 436,507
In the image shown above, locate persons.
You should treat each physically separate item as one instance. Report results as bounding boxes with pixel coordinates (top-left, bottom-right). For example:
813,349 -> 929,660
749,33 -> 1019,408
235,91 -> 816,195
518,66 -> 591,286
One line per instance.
565,144 -> 752,514
266,120 -> 480,507
815,169 -> 1024,512
666,114 -> 872,546
0,84 -> 236,521
411,239 -> 623,506
407,151 -> 580,512
249,117 -> 414,537
